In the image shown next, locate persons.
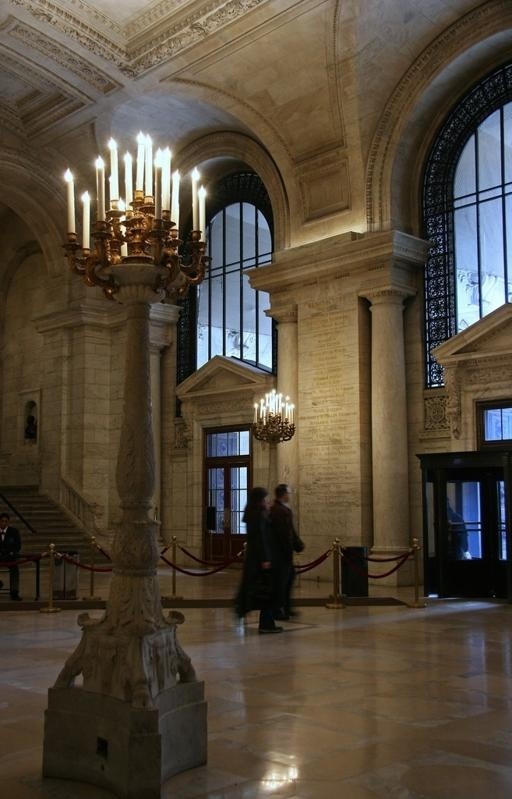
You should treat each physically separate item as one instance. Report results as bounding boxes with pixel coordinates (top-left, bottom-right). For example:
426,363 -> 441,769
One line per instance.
25,415 -> 37,439
0,512 -> 23,601
447,497 -> 468,560
258,484 -> 306,634
234,485 -> 290,624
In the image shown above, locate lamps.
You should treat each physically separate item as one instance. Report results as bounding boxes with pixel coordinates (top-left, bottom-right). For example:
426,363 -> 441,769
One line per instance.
43,120 -> 212,315
251,387 -> 301,443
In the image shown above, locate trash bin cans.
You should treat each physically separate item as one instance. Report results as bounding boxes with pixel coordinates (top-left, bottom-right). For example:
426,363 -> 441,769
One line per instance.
52,551 -> 80,601
341,546 -> 369,598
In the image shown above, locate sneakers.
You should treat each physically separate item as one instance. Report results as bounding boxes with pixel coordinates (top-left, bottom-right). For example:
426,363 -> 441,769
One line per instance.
259,626 -> 283,634
